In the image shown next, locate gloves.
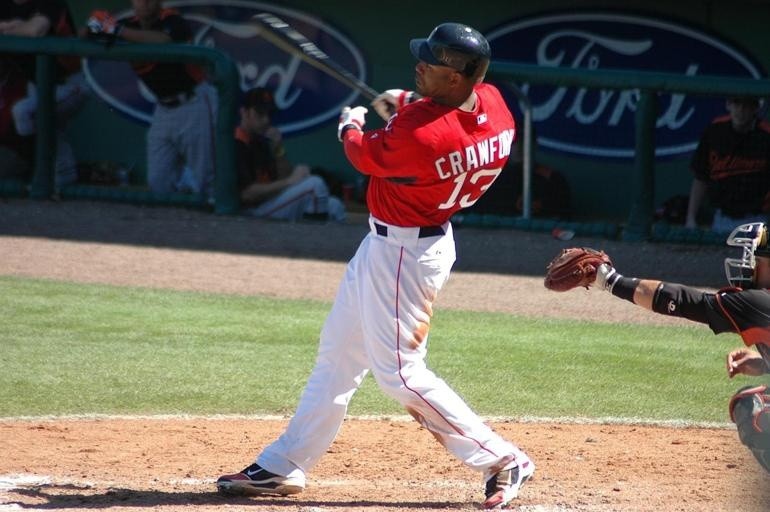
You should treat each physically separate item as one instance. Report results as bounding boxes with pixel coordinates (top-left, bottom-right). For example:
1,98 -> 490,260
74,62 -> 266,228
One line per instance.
86,10 -> 122,38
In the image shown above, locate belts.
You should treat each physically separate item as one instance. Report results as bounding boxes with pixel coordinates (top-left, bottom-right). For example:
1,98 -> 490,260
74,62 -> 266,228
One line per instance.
374,223 -> 445,237
160,91 -> 194,108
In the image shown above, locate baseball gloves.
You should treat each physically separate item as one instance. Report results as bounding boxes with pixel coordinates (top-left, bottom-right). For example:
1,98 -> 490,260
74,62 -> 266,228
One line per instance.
544,247 -> 612,291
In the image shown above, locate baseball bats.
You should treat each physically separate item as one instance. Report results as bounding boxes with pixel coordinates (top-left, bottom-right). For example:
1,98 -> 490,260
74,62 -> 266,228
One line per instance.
250,12 -> 396,116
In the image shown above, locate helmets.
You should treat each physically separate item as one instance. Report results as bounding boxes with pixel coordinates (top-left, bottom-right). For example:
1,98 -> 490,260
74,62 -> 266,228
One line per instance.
409,23 -> 491,84
723,222 -> 769,289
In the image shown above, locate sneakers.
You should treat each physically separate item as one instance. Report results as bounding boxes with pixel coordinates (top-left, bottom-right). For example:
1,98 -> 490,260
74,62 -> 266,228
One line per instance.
482,460 -> 537,508
216,463 -> 304,494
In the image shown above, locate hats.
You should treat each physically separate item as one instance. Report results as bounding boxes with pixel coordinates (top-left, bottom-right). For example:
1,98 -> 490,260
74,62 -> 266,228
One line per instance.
242,87 -> 279,114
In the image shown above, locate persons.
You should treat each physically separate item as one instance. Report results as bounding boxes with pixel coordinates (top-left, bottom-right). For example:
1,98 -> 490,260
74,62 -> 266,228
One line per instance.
233,86 -> 347,225
216,23 -> 539,510
78,0 -> 219,199
543,221 -> 769,473
0,1 -> 89,187
682,97 -> 769,233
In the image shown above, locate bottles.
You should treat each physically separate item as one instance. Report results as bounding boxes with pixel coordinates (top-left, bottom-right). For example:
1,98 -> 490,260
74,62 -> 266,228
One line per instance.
117,162 -> 130,187
